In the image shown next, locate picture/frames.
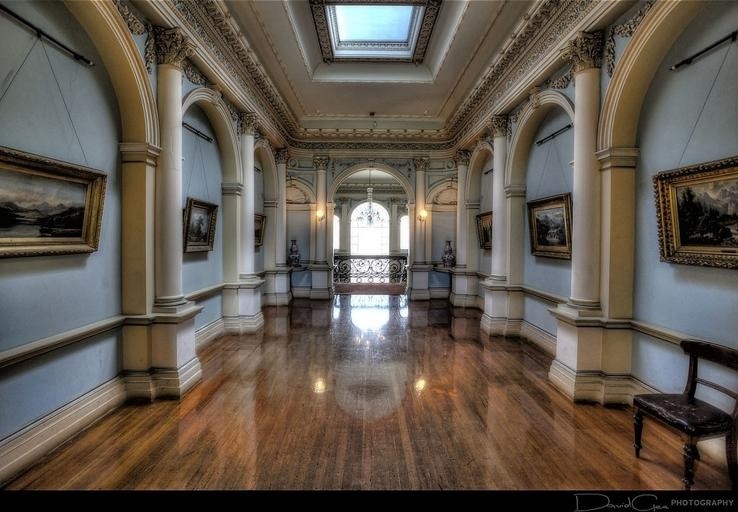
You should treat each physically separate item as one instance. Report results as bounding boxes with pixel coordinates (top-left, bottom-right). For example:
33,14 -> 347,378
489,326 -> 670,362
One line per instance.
183,197 -> 218,252
527,192 -> 573,259
254,212 -> 266,247
652,155 -> 738,268
0,146 -> 107,259
476,211 -> 492,249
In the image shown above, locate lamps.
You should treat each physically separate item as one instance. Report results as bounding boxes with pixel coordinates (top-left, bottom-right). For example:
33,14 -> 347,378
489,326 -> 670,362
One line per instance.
356,169 -> 385,224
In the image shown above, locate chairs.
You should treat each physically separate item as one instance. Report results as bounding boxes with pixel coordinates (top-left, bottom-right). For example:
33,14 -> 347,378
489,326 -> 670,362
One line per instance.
633,339 -> 737,489
